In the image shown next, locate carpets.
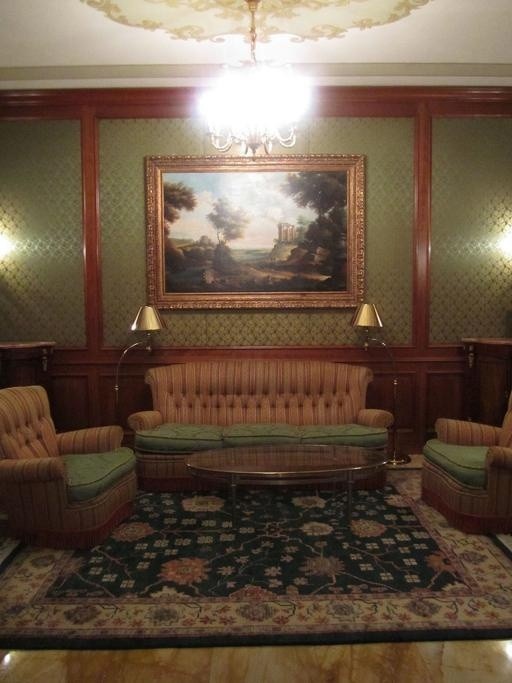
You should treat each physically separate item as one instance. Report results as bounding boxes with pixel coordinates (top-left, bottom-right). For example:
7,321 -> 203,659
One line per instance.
0,468 -> 512,650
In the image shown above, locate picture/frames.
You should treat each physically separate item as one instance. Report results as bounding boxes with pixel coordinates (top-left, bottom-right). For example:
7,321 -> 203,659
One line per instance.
143,152 -> 366,312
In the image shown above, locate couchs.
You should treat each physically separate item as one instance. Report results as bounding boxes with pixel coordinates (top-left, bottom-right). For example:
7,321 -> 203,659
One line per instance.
127,359 -> 395,491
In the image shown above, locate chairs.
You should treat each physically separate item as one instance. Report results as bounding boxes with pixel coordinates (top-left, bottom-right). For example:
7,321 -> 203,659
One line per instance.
422,389 -> 512,533
0,383 -> 142,549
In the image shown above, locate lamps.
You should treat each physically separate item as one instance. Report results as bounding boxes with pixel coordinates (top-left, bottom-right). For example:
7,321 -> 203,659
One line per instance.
86,0 -> 432,158
351,303 -> 412,466
114,306 -> 161,429
